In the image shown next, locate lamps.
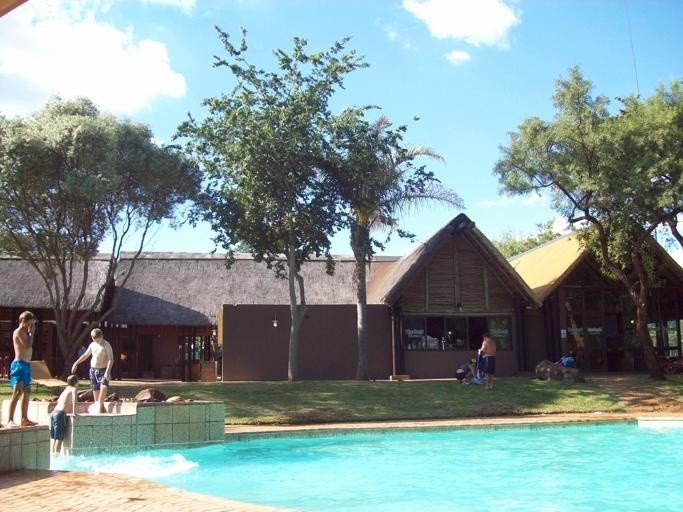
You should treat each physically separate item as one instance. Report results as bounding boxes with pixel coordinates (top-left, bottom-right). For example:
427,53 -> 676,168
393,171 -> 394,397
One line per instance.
272,315 -> 278,328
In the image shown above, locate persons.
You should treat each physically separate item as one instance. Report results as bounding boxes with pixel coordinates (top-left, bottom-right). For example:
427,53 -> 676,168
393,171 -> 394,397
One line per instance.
7,310 -> 38,427
50,328 -> 115,457
455,332 -> 497,390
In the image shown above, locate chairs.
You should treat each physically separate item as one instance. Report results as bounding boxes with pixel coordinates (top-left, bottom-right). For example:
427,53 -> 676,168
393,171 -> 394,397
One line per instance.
29,360 -> 68,396
